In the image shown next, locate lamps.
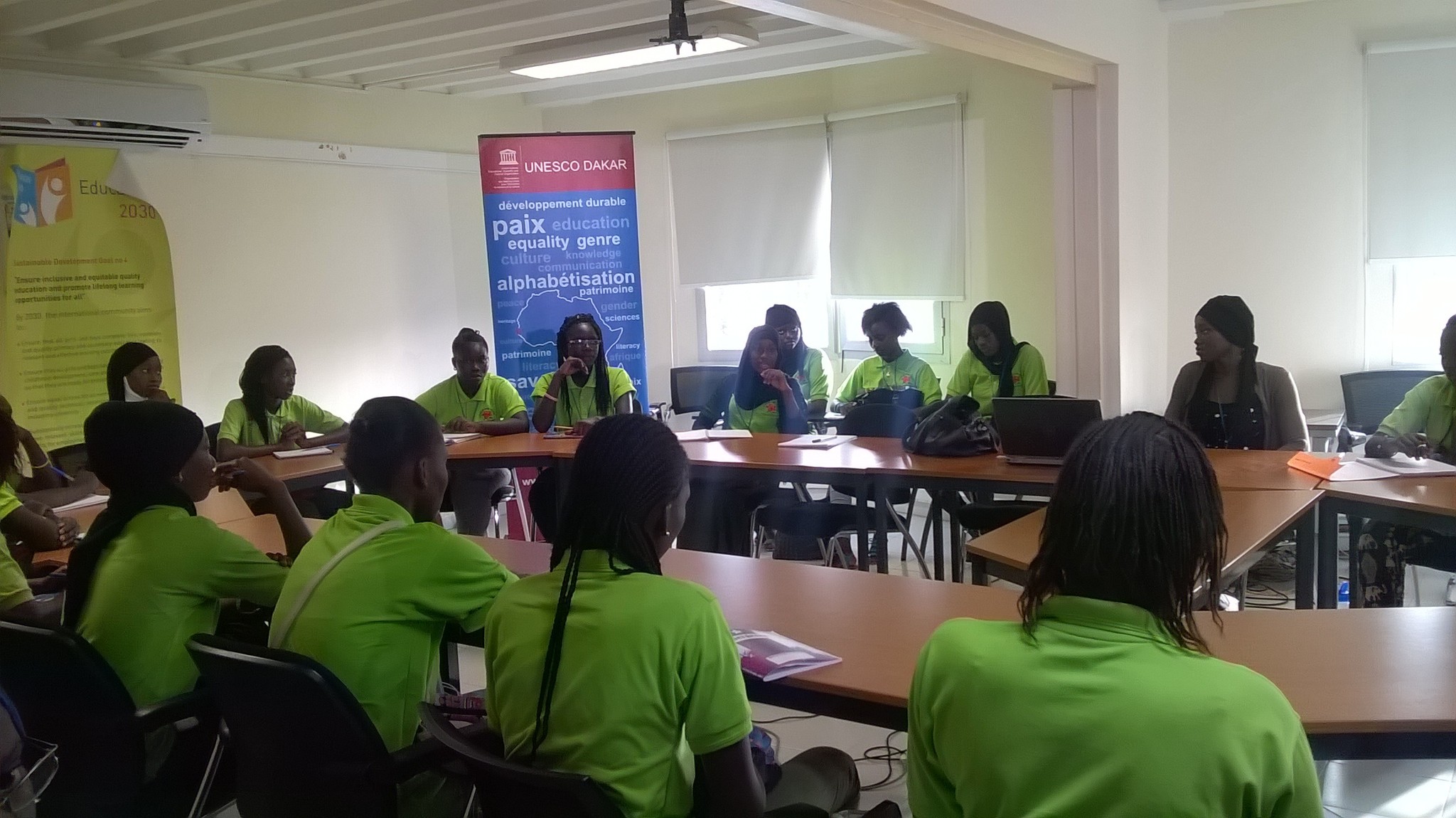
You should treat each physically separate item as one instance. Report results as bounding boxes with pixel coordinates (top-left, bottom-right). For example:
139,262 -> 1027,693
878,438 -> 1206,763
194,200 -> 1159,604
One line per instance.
499,17 -> 759,80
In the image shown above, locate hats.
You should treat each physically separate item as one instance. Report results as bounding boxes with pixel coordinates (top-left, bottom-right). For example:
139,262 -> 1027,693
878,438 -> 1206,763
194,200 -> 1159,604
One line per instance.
77,400 -> 203,501
107,341 -> 157,401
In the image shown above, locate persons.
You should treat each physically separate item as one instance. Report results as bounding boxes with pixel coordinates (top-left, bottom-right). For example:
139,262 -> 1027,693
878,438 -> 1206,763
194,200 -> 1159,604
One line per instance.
754,305 -> 830,551
1357,316 -> 1456,608
530,315 -> 636,546
480,412 -> 863,818
105,343 -> 176,405
673,326 -> 808,557
5,424 -> 99,510
908,417 -> 1321,818
923,302 -> 1049,561
0,410 -> 68,622
215,346 -> 358,519
409,328 -> 529,537
0,482 -> 81,551
829,303 -> 941,570
1158,295 -> 1311,453
265,397 -> 517,818
64,396 -> 314,818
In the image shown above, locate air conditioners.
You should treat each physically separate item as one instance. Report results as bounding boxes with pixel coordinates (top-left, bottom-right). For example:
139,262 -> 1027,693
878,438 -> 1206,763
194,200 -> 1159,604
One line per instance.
0,69 -> 211,152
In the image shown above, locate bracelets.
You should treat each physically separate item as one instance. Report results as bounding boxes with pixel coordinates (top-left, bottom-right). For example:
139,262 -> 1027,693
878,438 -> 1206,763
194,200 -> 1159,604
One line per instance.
834,402 -> 841,413
30,459 -> 50,469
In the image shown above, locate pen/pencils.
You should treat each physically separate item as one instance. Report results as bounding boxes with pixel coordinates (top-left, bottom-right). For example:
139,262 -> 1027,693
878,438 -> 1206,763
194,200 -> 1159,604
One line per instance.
49,466 -> 74,480
223,470 -> 244,476
552,426 -> 574,430
324,443 -> 342,447
812,436 -> 838,443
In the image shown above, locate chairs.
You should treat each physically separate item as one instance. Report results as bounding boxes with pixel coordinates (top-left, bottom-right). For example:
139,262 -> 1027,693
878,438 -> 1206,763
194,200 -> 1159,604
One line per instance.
0,620 -> 901,818
48,365 -> 1456,581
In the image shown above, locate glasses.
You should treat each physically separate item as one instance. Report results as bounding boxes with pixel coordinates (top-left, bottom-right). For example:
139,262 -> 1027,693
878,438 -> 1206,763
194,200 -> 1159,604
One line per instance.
565,339 -> 601,349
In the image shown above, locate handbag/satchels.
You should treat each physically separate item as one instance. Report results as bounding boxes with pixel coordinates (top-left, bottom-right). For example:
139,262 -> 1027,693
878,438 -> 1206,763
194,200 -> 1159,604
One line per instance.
902,395 -> 999,456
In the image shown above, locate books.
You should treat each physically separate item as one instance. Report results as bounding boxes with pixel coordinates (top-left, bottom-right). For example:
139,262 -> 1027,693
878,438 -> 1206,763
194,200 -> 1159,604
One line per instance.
725,628 -> 845,681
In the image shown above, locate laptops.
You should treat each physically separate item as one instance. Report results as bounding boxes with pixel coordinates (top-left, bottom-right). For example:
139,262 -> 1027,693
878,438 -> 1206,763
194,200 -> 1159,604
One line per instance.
998,397 -> 1101,472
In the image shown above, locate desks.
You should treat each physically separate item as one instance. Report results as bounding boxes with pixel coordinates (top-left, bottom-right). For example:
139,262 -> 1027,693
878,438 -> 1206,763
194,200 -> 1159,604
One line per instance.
31,433 -> 1456,763
